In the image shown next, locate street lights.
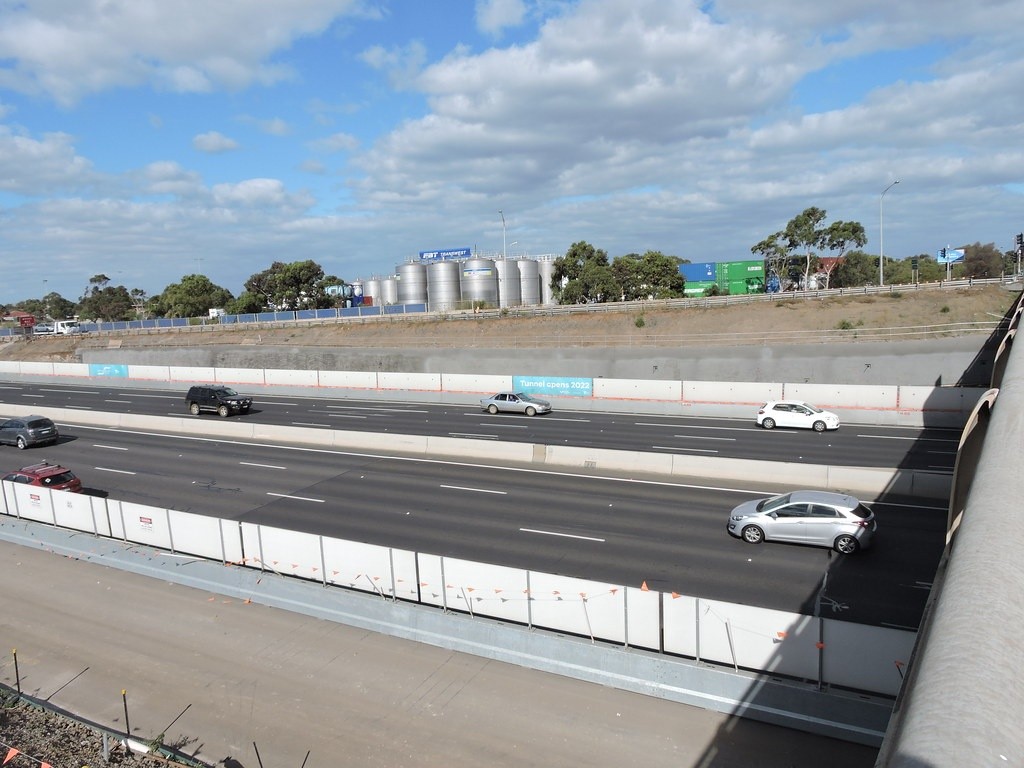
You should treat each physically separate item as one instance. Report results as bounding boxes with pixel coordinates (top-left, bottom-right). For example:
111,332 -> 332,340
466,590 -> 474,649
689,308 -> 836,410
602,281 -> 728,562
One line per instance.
498,209 -> 508,309
880,180 -> 900,287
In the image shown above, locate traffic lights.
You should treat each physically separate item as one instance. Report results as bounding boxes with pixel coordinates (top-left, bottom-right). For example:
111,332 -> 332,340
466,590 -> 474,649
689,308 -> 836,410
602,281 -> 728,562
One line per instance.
1013,253 -> 1018,263
941,248 -> 945,258
875,258 -> 879,267
1016,232 -> 1023,244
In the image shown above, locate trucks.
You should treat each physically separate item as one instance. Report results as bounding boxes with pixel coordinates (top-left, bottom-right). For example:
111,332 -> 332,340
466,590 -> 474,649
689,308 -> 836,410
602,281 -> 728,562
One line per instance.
32,320 -> 83,337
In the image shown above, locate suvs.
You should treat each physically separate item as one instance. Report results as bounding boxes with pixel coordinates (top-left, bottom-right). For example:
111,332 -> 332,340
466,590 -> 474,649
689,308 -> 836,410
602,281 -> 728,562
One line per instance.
0,460 -> 86,495
184,383 -> 253,418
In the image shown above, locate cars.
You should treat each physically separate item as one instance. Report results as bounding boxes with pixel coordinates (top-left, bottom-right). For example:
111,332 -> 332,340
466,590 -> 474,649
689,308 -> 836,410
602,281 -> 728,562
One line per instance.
756,400 -> 841,433
0,414 -> 60,451
726,489 -> 879,558
35,322 -> 54,332
479,390 -> 553,417
